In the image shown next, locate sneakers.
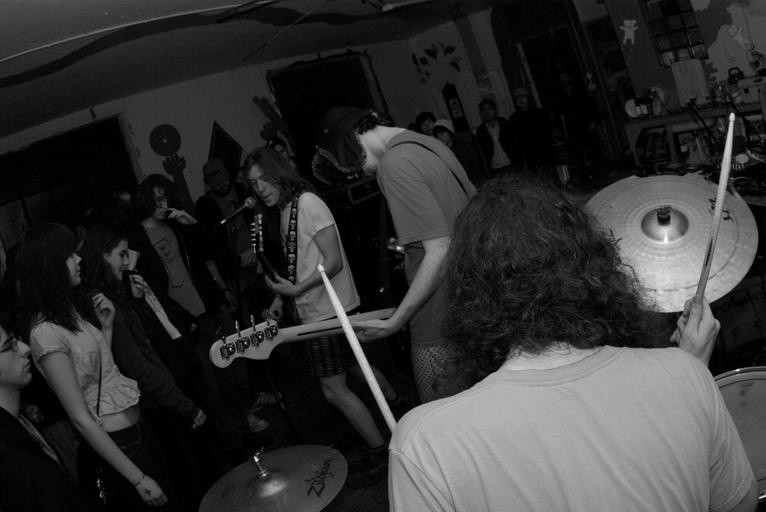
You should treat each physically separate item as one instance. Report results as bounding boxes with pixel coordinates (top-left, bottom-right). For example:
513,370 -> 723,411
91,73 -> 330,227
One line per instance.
247,413 -> 270,432
367,396 -> 405,413
348,447 -> 391,487
249,392 -> 281,414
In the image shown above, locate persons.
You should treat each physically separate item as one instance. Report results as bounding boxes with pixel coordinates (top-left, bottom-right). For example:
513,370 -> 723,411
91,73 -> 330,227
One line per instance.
86,223 -> 214,511
1,323 -> 83,511
194,158 -> 284,327
432,119 -> 473,184
29,222 -> 169,511
416,110 -> 436,137
319,108 -> 479,405
388,173 -> 757,512
130,174 -> 270,434
244,147 -> 388,483
508,87 -> 554,174
271,138 -> 316,195
474,99 -> 520,175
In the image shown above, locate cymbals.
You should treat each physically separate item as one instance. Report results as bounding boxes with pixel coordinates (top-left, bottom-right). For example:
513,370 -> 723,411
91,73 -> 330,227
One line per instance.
582,170 -> 760,314
198,444 -> 348,512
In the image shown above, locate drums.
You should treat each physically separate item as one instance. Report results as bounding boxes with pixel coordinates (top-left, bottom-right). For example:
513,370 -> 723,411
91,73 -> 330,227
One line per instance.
711,365 -> 766,512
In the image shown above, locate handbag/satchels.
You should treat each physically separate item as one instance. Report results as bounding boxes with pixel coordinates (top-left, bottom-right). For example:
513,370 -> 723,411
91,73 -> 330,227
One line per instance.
57,329 -> 103,474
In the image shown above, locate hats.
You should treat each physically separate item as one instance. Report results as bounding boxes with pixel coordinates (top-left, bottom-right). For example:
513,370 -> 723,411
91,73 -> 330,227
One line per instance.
432,119 -> 455,134
315,107 -> 375,172
478,98 -> 496,107
512,88 -> 528,97
204,158 -> 228,186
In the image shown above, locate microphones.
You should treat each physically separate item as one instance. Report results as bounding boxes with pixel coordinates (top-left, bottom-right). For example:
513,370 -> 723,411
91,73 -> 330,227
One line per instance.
219,196 -> 257,225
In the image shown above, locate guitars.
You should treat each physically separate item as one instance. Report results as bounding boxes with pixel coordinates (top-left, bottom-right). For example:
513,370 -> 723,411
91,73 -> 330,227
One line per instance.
210,305 -> 408,368
247,213 -> 301,324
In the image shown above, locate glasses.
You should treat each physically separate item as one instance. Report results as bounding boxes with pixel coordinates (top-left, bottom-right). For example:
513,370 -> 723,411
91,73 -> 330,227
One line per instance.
0,336 -> 23,354
